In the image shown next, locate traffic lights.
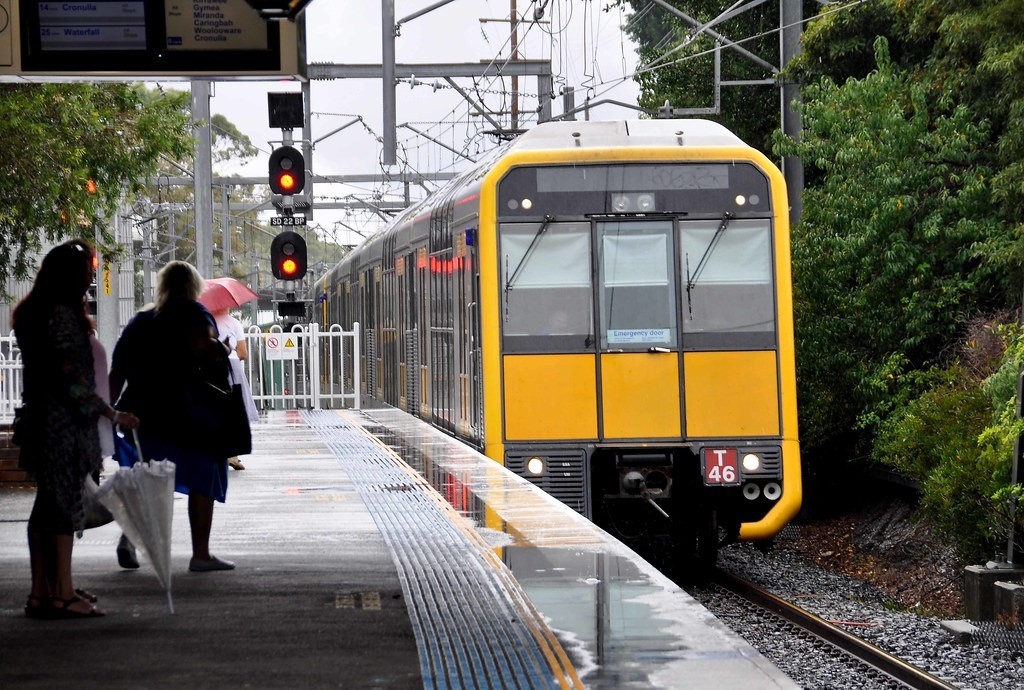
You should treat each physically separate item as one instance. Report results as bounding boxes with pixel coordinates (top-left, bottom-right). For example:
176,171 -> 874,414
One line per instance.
270,232 -> 307,282
268,147 -> 305,193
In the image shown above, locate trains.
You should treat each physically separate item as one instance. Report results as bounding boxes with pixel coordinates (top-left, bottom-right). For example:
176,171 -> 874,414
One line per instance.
310,77 -> 802,576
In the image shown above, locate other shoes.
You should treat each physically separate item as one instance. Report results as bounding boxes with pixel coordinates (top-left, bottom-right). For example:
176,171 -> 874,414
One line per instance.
116,537 -> 141,569
227,457 -> 246,470
188,556 -> 236,572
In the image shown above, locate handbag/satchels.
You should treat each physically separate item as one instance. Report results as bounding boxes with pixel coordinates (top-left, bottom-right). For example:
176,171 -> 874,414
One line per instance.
74,472 -> 113,532
193,338 -> 253,459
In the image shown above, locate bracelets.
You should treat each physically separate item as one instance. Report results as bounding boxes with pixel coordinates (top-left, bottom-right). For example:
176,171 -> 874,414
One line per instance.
113,410 -> 120,421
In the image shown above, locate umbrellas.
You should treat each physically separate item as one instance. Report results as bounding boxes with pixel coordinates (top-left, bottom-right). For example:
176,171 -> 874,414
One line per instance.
95,419 -> 176,616
196,276 -> 260,311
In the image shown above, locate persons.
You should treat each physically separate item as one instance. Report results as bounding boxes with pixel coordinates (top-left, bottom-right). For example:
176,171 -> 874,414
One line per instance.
10,239 -> 139,617
211,307 -> 260,471
108,262 -> 237,571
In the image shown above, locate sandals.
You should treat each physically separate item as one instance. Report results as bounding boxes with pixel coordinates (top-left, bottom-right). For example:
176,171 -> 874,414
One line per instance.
44,595 -> 106,619
25,593 -> 46,619
